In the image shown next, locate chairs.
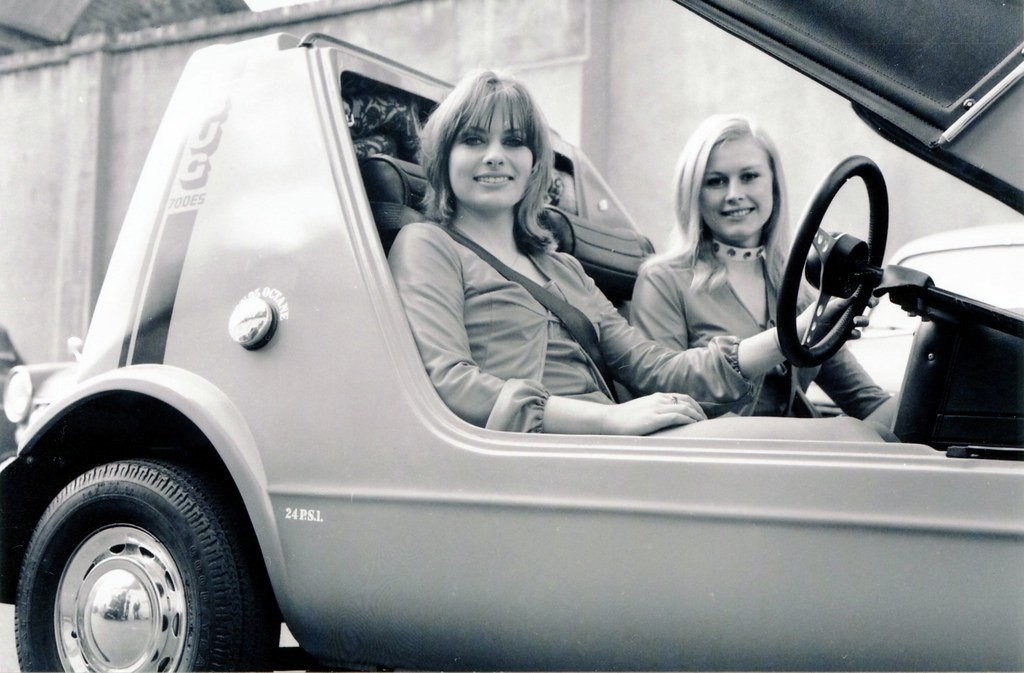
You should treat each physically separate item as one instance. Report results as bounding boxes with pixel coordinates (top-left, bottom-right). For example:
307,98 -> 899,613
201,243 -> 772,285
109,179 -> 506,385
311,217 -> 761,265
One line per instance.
536,205 -> 654,398
362,154 -> 428,259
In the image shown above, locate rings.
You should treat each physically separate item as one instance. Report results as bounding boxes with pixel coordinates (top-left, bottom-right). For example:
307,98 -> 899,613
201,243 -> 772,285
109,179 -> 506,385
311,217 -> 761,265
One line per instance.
672,397 -> 677,404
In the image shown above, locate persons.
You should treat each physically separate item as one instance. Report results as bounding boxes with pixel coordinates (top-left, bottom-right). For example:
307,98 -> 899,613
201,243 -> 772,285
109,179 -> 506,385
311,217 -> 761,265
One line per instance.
388,68 -> 879,435
628,113 -> 890,421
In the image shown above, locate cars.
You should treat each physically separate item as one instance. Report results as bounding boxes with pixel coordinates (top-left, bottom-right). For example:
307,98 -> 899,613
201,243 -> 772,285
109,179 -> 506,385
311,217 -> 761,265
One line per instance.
0,0 -> 1024,672
